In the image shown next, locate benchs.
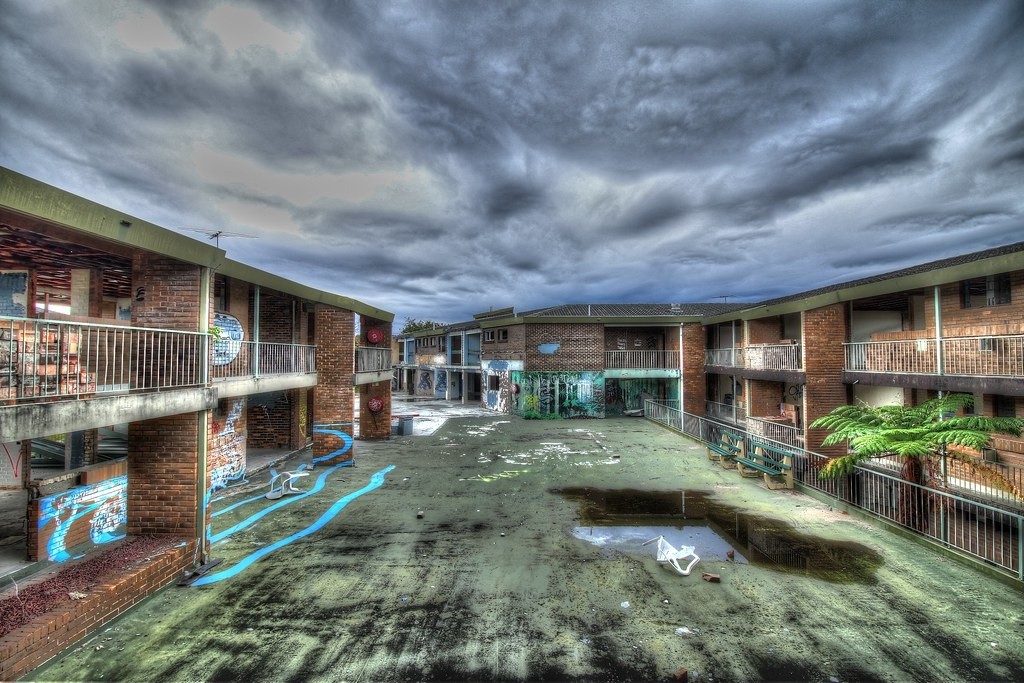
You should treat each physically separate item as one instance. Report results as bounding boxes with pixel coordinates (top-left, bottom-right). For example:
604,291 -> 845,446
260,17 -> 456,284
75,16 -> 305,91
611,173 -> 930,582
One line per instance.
734,441 -> 795,490
704,430 -> 745,470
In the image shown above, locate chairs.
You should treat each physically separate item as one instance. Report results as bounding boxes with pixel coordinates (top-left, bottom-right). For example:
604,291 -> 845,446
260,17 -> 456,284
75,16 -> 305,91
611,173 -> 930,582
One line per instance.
265,469 -> 310,499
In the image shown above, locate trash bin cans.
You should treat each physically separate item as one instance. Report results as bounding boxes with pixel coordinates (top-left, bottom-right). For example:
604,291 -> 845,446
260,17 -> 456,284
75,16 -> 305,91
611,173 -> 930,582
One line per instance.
399,416 -> 413,436
